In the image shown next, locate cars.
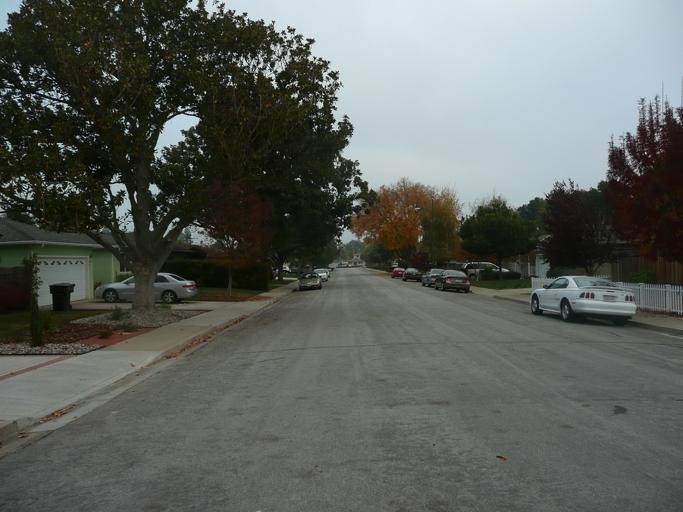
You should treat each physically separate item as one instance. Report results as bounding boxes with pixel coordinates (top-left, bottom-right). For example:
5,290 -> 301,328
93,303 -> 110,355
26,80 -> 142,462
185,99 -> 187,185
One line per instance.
390,268 -> 405,278
529,275 -> 637,325
420,268 -> 444,288
297,267 -> 330,290
402,268 -> 421,281
93,272 -> 197,304
434,270 -> 470,293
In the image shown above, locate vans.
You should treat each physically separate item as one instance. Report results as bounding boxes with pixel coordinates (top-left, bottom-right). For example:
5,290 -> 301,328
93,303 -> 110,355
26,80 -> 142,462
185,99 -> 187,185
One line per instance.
461,262 -> 510,281
